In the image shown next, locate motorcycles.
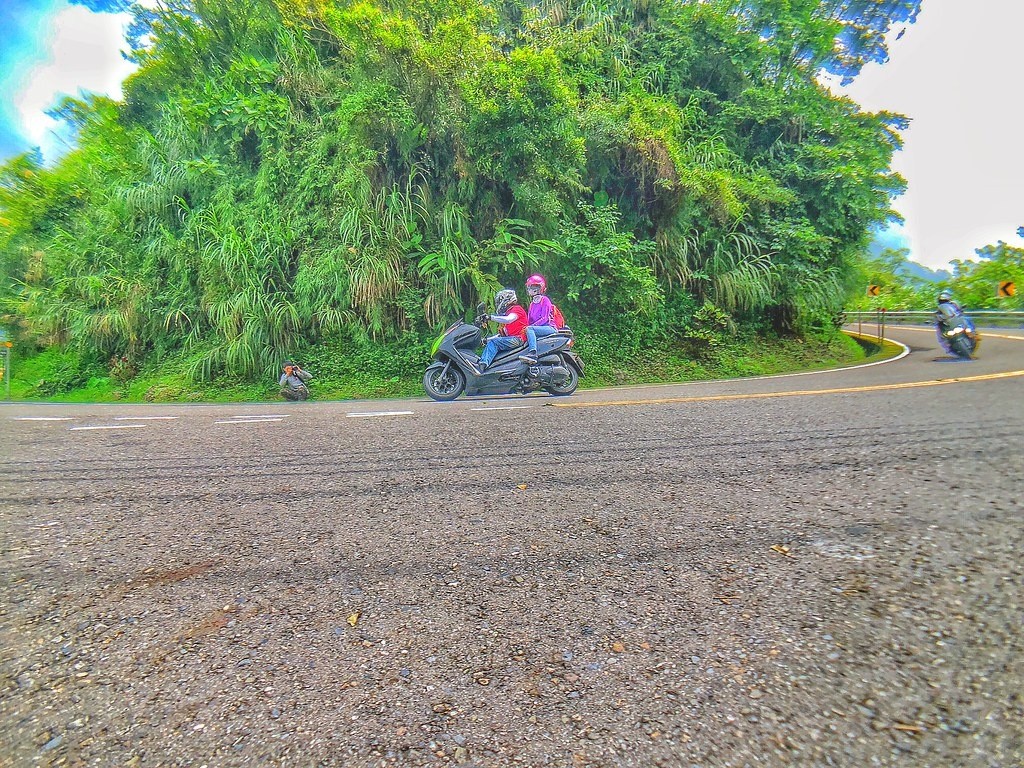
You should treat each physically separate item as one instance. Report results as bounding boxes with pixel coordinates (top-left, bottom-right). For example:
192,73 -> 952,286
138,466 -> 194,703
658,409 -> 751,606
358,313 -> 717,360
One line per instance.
423,302 -> 586,401
925,302 -> 977,360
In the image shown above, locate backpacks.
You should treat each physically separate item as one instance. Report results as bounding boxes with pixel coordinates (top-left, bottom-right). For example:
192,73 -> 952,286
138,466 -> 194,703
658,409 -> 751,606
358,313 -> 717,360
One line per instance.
541,296 -> 564,329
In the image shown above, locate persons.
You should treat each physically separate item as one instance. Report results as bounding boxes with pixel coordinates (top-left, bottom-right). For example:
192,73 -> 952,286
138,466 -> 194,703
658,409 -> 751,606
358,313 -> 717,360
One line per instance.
933,290 -> 980,354
519,274 -> 558,364
466,289 -> 530,374
278,361 -> 313,404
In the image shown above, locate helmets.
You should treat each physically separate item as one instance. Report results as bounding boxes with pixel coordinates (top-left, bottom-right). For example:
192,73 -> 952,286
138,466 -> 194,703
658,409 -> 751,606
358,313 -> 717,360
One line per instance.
938,294 -> 949,303
495,289 -> 517,314
525,274 -> 545,293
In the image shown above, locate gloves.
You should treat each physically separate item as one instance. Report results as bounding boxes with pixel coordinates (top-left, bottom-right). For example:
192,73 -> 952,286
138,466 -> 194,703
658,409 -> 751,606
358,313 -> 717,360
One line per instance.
482,313 -> 490,320
481,338 -> 487,346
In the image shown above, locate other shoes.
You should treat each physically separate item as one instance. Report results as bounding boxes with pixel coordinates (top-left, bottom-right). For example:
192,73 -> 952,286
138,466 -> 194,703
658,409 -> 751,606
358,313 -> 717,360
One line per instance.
466,359 -> 482,375
519,354 -> 538,364
